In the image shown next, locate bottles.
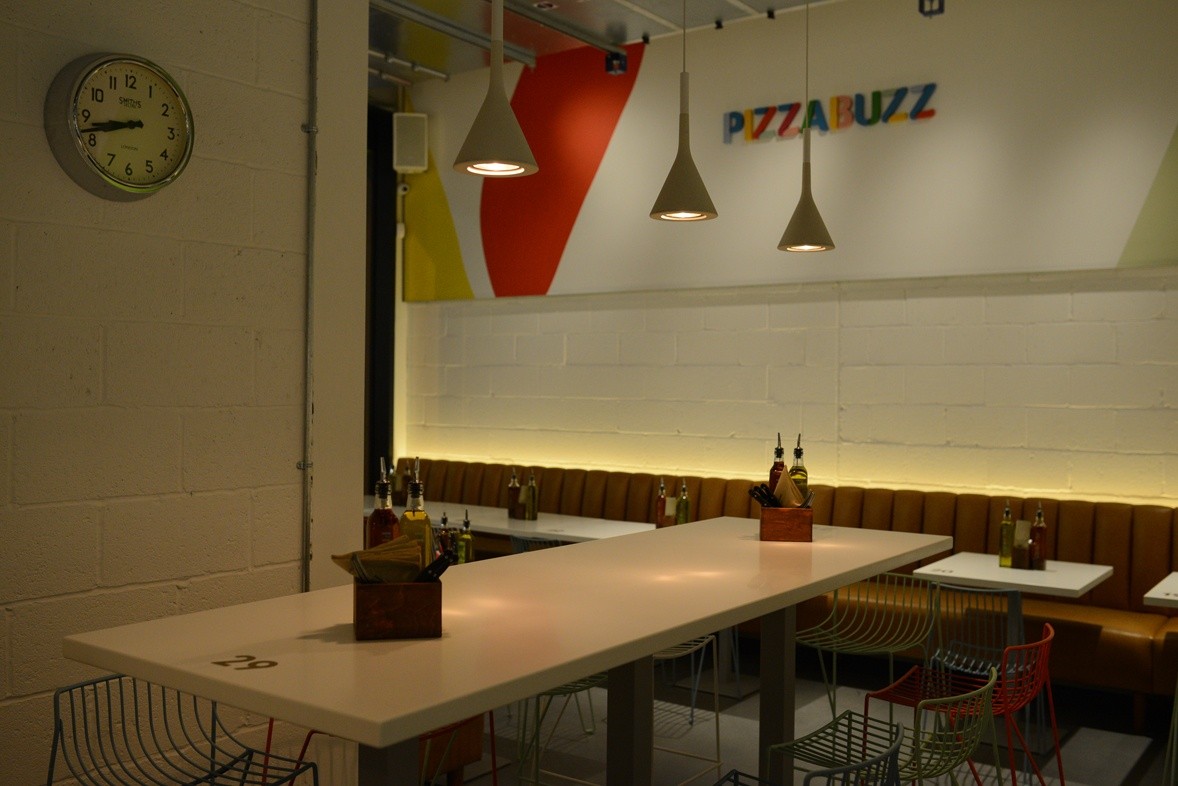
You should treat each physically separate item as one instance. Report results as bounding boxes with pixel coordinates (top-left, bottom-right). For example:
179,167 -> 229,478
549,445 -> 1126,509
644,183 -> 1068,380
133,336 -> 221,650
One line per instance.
1029,499 -> 1047,570
999,497 -> 1014,567
655,477 -> 668,529
675,478 -> 691,525
526,467 -> 538,520
768,432 -> 786,493
789,434 -> 808,499
368,456 -> 473,567
508,466 -> 520,517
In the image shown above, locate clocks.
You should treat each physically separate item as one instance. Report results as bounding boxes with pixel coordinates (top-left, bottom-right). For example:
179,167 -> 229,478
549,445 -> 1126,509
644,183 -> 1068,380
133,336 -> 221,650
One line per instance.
43,51 -> 195,202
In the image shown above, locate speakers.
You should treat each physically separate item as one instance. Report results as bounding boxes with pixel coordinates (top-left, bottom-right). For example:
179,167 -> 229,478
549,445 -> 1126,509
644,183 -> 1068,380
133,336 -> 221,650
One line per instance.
393,112 -> 430,174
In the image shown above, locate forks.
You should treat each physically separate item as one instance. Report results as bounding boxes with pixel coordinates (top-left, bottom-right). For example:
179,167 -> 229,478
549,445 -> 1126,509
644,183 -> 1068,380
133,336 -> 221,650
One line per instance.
797,489 -> 815,508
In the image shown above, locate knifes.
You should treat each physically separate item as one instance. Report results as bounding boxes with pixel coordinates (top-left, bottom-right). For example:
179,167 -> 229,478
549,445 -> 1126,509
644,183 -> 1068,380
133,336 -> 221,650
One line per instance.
749,483 -> 785,507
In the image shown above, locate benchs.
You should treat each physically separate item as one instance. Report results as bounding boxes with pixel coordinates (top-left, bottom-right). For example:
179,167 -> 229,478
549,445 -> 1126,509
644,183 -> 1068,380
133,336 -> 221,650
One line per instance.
392,457 -> 1178,734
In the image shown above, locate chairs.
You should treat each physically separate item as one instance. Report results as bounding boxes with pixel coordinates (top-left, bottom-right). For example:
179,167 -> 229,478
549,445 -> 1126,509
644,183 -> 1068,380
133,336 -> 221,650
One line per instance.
419,674 -> 607,786
860,623 -> 1066,786
652,634 -> 723,786
44,672 -> 320,786
710,722 -> 905,786
764,667 -> 1004,786
929,581 -> 1049,786
795,571 -> 948,748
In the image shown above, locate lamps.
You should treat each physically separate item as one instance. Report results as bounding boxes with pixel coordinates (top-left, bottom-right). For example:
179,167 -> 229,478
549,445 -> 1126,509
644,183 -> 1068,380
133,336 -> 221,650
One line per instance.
650,70 -> 719,222
777,127 -> 836,252
452,0 -> 539,178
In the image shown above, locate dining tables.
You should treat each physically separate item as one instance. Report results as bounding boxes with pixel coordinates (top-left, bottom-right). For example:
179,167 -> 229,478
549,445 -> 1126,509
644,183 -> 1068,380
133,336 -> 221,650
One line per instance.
362,494 -> 656,543
58,515 -> 954,786
1144,572 -> 1178,609
913,551 -> 1115,756
